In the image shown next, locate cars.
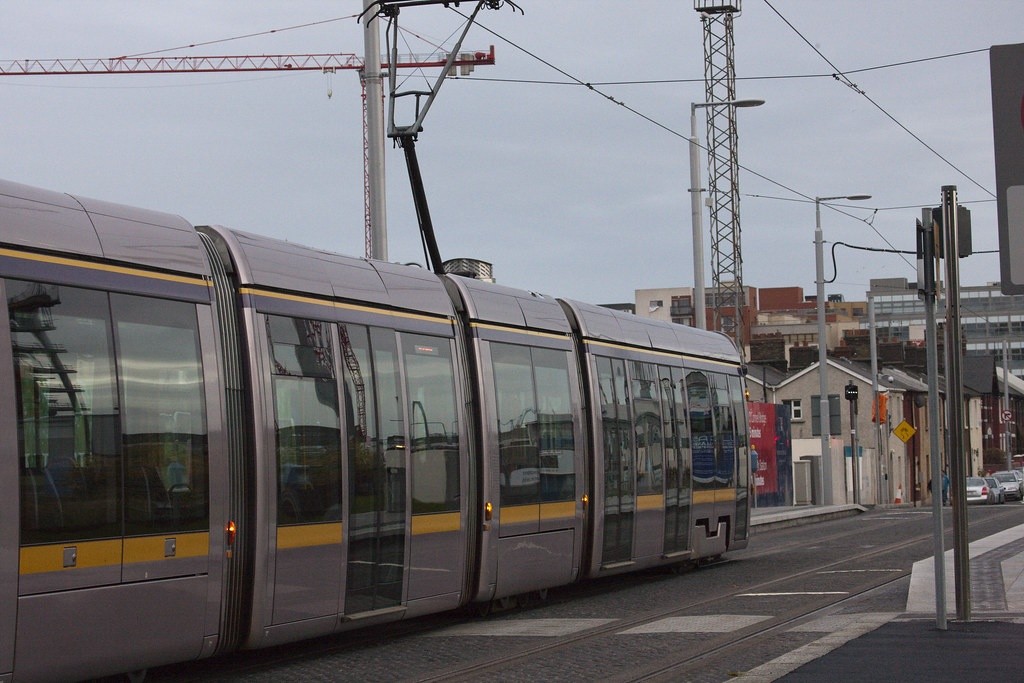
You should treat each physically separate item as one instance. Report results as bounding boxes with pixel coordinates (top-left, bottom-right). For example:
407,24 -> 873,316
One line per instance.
984,476 -> 1006,504
962,476 -> 991,504
991,467 -> 1024,500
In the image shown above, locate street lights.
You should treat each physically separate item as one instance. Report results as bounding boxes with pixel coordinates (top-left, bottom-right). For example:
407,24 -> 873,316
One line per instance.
689,99 -> 765,333
813,194 -> 873,507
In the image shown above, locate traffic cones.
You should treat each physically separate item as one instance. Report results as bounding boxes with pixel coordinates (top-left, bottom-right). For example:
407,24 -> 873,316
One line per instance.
893,484 -> 903,503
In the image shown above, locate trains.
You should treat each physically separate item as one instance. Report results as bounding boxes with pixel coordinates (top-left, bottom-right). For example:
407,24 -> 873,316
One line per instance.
1,179 -> 756,683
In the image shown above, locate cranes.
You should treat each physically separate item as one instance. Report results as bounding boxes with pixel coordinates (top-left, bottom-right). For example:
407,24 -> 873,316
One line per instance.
0,45 -> 497,258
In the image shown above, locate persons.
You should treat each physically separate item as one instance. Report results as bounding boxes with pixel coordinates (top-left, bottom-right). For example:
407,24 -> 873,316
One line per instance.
927,479 -> 933,494
941,469 -> 950,506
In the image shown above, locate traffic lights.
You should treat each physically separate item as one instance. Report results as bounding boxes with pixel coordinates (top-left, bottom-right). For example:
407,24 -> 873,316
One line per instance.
844,384 -> 859,401
